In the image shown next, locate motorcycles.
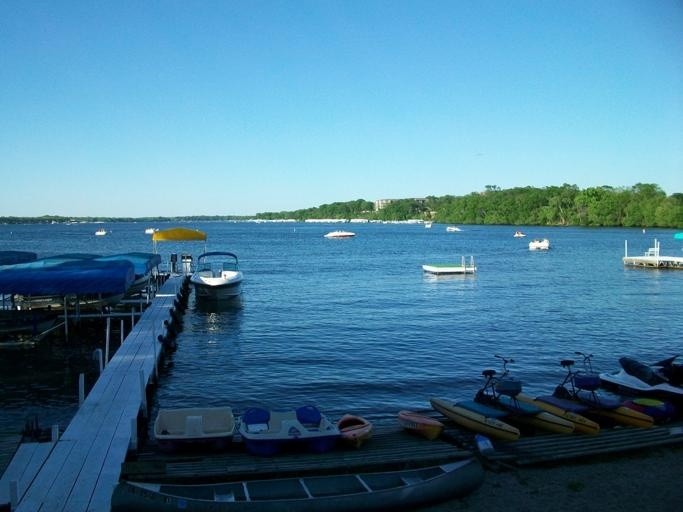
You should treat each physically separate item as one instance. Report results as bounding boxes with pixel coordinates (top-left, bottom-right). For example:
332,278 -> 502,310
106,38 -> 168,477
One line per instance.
598,351 -> 683,404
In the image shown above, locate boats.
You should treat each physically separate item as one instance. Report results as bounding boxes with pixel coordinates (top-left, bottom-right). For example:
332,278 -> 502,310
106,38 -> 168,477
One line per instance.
152,404 -> 443,461
447,227 -> 461,232
425,223 -> 432,228
422,256 -> 477,274
324,229 -> 356,238
513,231 -> 526,237
94,223 -> 112,235
190,251 -> 244,299
13,273 -> 152,312
528,238 -> 549,250
144,226 -> 160,234
